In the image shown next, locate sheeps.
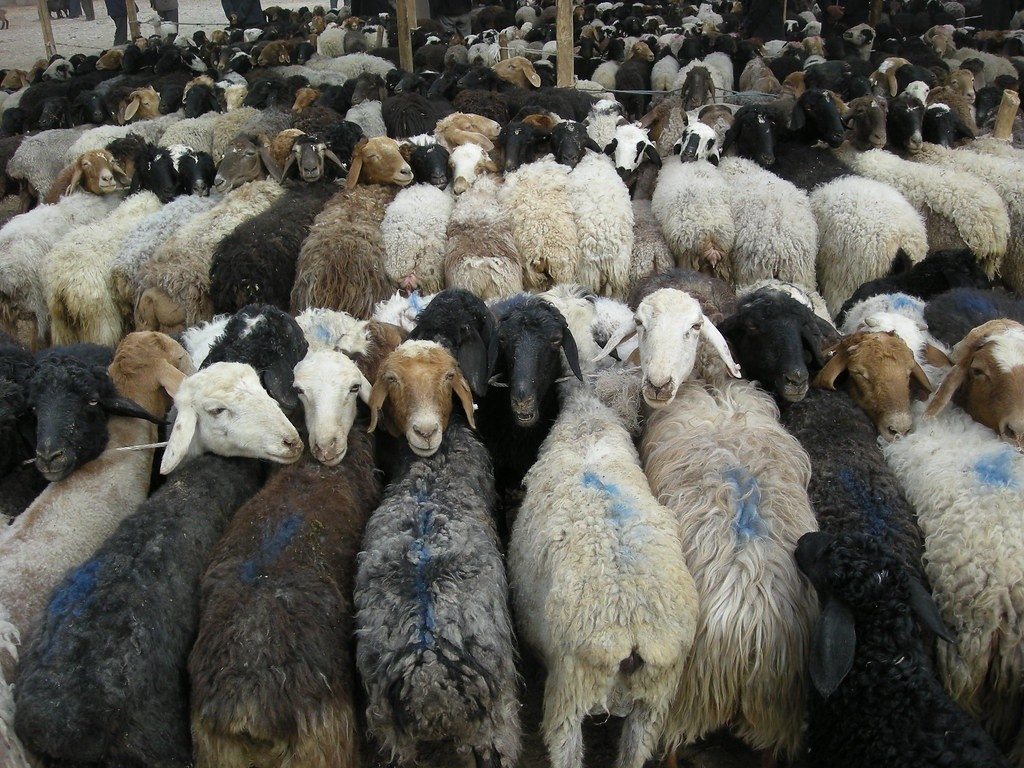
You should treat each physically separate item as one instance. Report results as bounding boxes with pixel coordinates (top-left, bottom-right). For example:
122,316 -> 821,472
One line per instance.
0,0 -> 1024,768
